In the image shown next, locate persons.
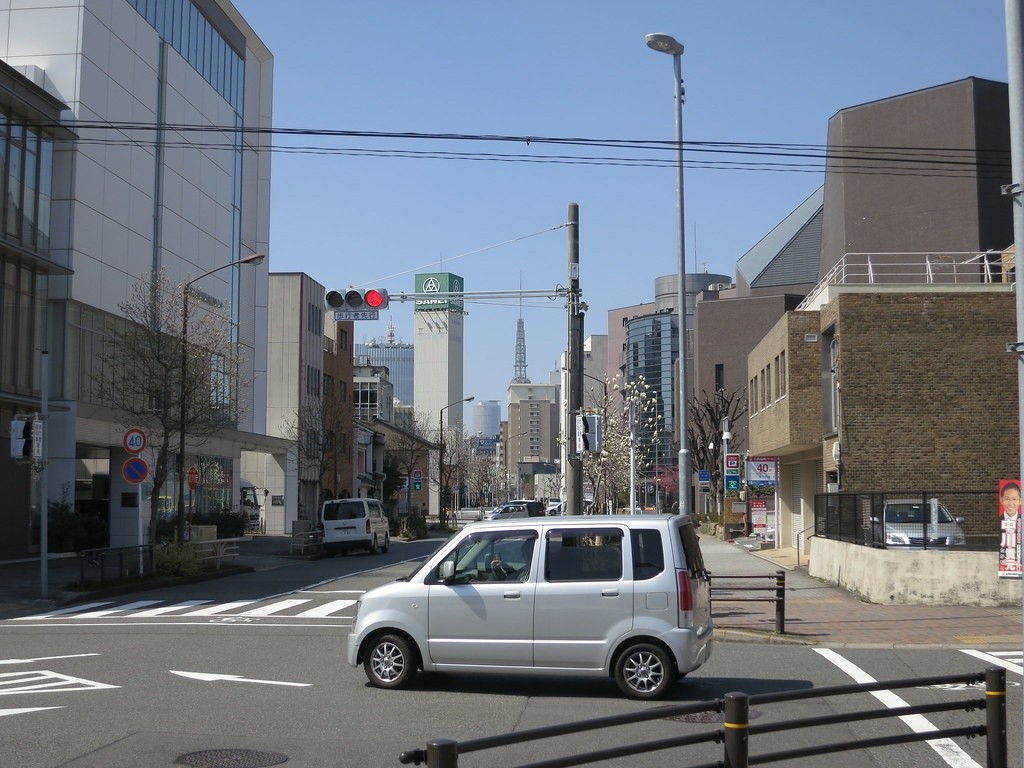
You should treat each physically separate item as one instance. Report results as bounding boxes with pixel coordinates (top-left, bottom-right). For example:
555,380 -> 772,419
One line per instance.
999,483 -> 1022,527
422,503 -> 427,510
490,538 -> 534,582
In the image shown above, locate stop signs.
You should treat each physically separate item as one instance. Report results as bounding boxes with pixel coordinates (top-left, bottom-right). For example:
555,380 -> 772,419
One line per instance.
188,467 -> 200,489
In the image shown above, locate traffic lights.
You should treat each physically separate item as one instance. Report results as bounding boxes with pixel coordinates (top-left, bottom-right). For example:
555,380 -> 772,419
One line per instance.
9,419 -> 32,458
324,287 -> 389,311
580,414 -> 603,453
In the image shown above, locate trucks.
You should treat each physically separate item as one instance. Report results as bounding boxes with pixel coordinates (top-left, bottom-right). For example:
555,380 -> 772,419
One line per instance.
239,478 -> 268,533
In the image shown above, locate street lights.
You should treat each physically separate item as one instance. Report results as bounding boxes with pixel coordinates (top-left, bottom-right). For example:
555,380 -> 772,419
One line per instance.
708,436 -> 717,514
504,431 -> 527,503
178,253 -> 268,542
721,417 -> 733,502
562,368 -> 607,514
438,397 -> 473,524
645,32 -> 693,518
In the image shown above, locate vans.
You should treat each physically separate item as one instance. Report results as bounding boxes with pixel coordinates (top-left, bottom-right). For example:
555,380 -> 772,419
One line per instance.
158,496 -> 174,519
546,497 -> 560,507
319,498 -> 390,557
183,493 -> 196,512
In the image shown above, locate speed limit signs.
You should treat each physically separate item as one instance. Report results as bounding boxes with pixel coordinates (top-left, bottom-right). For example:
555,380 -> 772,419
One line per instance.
123,429 -> 147,455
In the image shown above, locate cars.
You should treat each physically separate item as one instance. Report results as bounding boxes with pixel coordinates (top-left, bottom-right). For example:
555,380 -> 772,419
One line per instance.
344,514 -> 715,700
508,500 -> 544,517
867,499 -> 968,551
482,504 -> 530,520
546,499 -> 594,515
452,507 -> 482,520
